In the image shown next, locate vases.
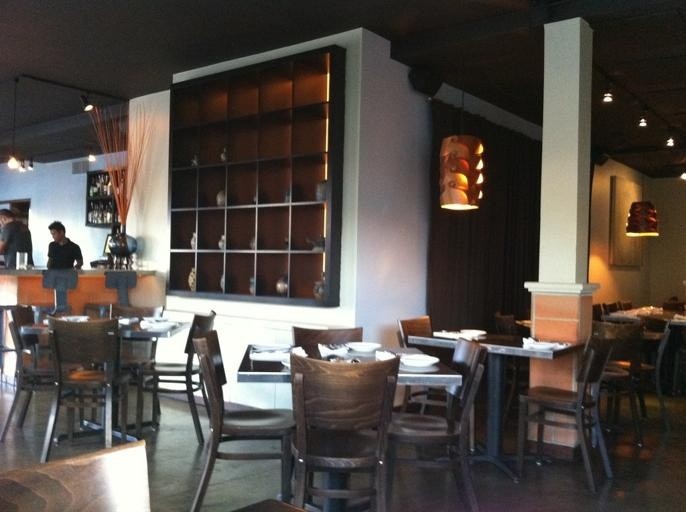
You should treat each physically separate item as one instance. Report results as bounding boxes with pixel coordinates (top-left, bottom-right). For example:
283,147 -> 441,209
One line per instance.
107,232 -> 138,257
245,235 -> 288,298
186,145 -> 228,296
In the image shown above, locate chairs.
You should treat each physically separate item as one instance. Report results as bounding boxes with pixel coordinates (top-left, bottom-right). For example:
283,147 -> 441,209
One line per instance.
0,267 -> 686,512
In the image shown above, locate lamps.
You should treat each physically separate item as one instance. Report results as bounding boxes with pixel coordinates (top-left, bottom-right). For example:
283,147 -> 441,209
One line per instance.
19,157 -> 34,172
5,75 -> 21,169
597,67 -> 677,150
437,77 -> 484,212
621,135 -> 660,240
75,87 -> 96,114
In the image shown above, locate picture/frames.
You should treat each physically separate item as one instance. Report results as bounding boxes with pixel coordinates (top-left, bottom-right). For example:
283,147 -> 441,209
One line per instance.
606,174 -> 647,270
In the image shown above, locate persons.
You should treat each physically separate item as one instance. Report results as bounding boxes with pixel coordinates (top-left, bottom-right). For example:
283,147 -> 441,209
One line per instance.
0,209 -> 35,269
47,221 -> 83,270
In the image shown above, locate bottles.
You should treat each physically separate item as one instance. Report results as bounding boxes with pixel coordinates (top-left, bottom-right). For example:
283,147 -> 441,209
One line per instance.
187,268 -> 195,291
218,234 -> 224,250
87,201 -> 121,225
220,148 -> 227,162
312,280 -> 325,300
189,232 -> 196,249
88,172 -> 117,197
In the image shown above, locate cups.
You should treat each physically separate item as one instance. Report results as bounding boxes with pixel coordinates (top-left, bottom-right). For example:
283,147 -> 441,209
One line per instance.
15,251 -> 27,271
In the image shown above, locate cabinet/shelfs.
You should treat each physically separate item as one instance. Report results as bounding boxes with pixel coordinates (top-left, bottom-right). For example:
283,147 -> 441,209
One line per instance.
81,167 -> 124,229
162,42 -> 347,312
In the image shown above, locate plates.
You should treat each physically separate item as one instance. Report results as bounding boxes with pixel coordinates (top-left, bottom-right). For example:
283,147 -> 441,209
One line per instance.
248,341 -> 440,368
42,316 -> 176,332
434,329 -> 558,350
401,365 -> 440,374
